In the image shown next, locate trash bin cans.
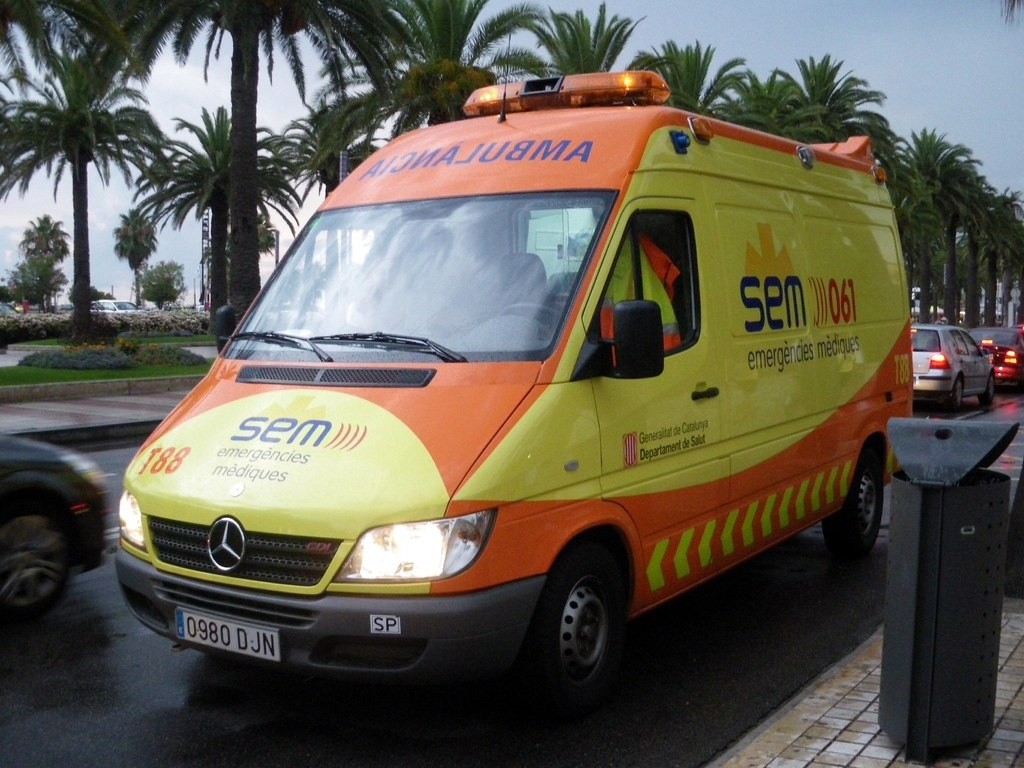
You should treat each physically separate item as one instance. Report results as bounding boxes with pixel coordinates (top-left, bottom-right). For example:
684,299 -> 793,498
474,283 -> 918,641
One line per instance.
875,415 -> 1019,762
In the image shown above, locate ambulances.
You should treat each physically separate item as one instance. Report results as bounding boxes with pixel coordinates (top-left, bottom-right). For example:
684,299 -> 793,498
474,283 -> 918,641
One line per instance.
113,70 -> 916,732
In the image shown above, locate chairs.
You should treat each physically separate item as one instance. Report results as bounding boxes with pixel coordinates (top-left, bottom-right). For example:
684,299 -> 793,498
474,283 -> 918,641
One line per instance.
496,254 -> 550,323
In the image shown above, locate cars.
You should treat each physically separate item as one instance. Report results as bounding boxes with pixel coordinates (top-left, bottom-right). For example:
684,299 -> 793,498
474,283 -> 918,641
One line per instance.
1,305 -> 19,315
92,300 -> 146,314
0,434 -> 112,628
911,324 -> 996,414
967,326 -> 1024,394
